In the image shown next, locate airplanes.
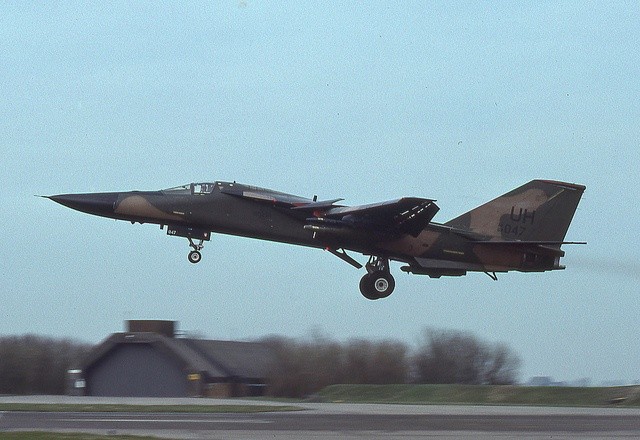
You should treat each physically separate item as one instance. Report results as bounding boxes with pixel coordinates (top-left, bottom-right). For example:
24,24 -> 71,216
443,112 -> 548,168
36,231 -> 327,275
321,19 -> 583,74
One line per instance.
32,178 -> 589,300
